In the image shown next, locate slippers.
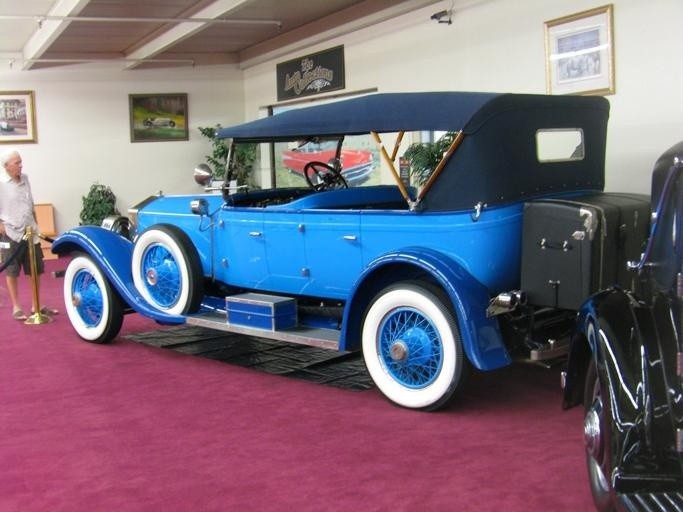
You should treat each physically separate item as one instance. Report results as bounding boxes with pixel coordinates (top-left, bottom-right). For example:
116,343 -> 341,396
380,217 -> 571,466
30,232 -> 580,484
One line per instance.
10,305 -> 60,320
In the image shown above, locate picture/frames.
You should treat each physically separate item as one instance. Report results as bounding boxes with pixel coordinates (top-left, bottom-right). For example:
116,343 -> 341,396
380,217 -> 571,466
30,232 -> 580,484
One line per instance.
542,3 -> 616,98
126,91 -> 190,144
0,90 -> 37,146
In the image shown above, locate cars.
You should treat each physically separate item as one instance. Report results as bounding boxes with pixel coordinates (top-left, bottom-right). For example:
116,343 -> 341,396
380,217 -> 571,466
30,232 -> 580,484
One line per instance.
283,142 -> 372,186
559,142 -> 683,511
50,88 -> 653,412
142,117 -> 174,127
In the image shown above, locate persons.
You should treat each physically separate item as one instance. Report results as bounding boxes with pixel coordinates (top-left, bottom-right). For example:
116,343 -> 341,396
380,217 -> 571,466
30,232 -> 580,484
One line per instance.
0,148 -> 60,321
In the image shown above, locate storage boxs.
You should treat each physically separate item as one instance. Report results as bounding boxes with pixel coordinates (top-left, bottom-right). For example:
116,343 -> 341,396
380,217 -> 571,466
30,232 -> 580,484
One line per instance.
224,293 -> 302,332
